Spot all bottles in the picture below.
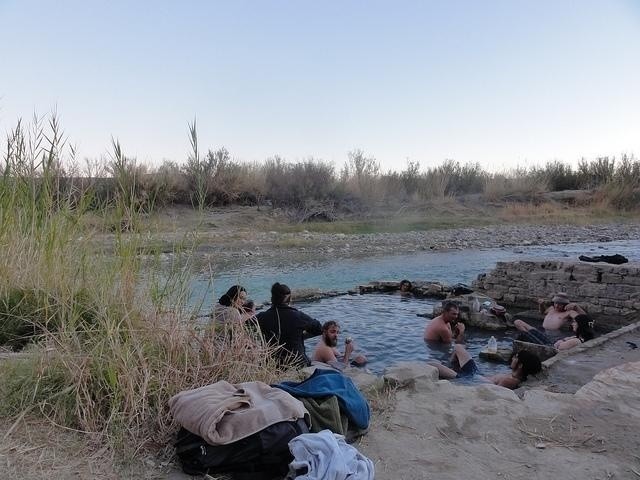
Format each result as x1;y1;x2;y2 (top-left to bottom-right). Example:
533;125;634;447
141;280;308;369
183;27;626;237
487;335;498;355
472;298;480;313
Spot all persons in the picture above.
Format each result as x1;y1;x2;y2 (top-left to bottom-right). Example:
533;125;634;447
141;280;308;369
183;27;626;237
516;314;594;351
513;291;587;334
239;299;257;321
313;320;355;365
422;301;466;344
426;342;542;390
393;280;414;296
199;282;248;354
245;282;325;373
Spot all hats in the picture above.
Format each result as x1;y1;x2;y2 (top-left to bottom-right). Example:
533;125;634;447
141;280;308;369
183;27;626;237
551;291;570;304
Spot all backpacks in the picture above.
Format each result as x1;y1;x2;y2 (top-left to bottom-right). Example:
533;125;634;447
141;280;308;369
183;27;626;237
174;416;314;480
